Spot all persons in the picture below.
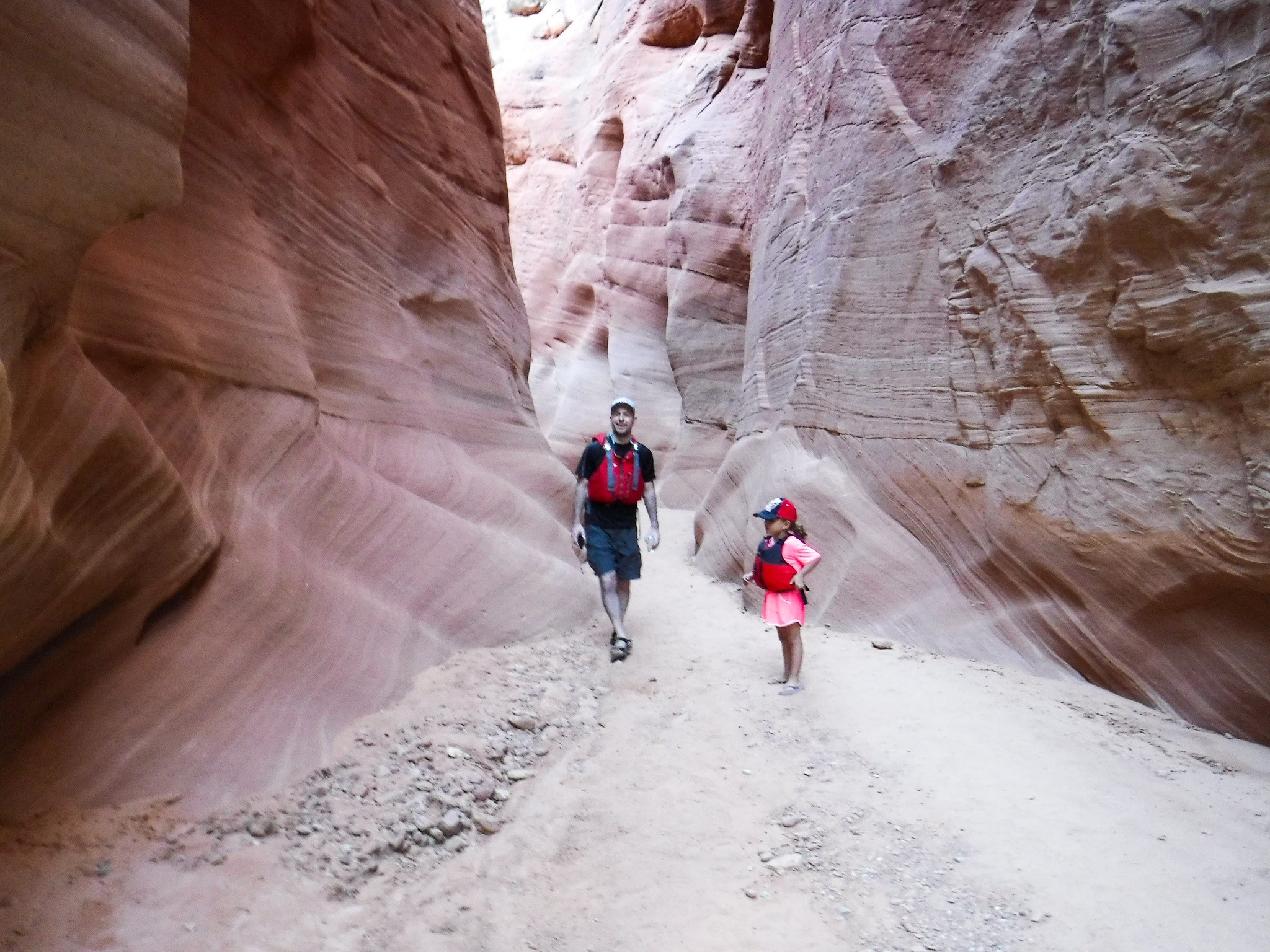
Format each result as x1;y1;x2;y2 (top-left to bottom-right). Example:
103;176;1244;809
743;498;823;697
571;397;660;662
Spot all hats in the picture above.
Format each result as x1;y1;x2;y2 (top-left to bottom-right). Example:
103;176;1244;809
753;497;797;520
610;397;635;414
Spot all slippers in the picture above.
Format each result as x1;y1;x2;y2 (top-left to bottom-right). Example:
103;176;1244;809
778;684;804;696
768;678;787;685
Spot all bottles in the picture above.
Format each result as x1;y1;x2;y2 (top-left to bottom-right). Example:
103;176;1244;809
646;535;655;553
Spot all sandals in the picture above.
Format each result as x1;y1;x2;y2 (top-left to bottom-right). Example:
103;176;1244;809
610;636;632;662
610;631;616;646
